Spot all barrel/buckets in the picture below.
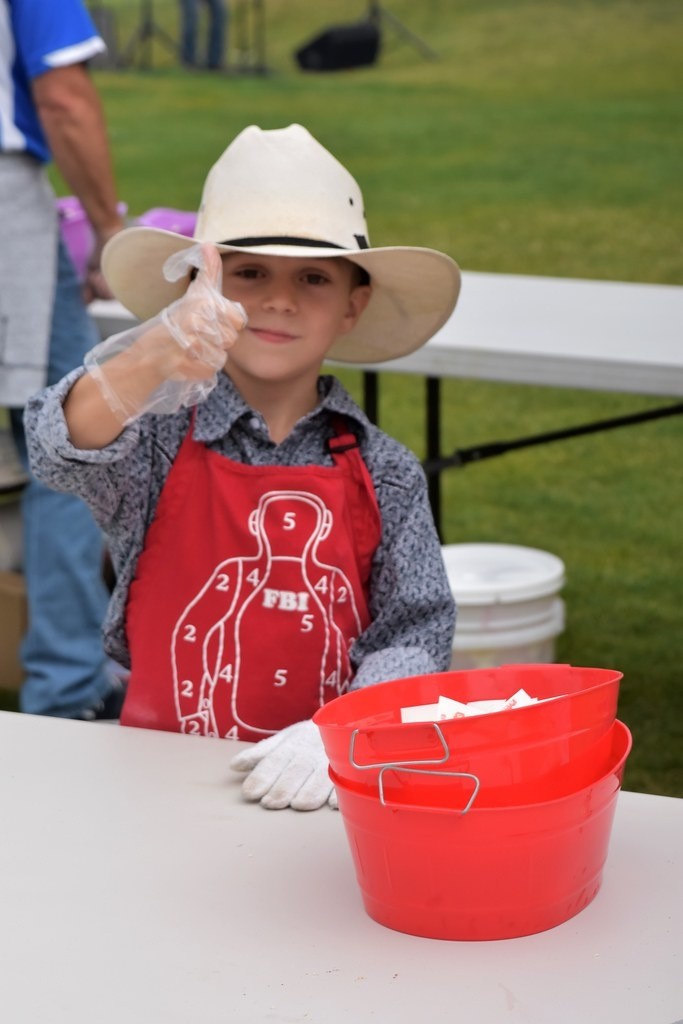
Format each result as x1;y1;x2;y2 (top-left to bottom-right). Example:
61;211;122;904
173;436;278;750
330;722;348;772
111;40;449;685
312;541;637;943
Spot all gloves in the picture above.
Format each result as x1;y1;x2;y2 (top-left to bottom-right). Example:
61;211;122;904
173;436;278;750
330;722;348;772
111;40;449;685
229;718;341;810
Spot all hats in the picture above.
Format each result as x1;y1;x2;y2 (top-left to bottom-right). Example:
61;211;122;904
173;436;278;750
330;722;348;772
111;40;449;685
100;123;463;365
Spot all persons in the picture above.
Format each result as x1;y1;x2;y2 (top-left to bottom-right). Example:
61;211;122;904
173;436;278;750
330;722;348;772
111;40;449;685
20;122;461;813
0;0;129;718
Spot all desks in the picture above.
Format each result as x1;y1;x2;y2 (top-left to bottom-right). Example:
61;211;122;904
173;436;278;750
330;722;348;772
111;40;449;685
82;266;682;553
0;709;682;1020
